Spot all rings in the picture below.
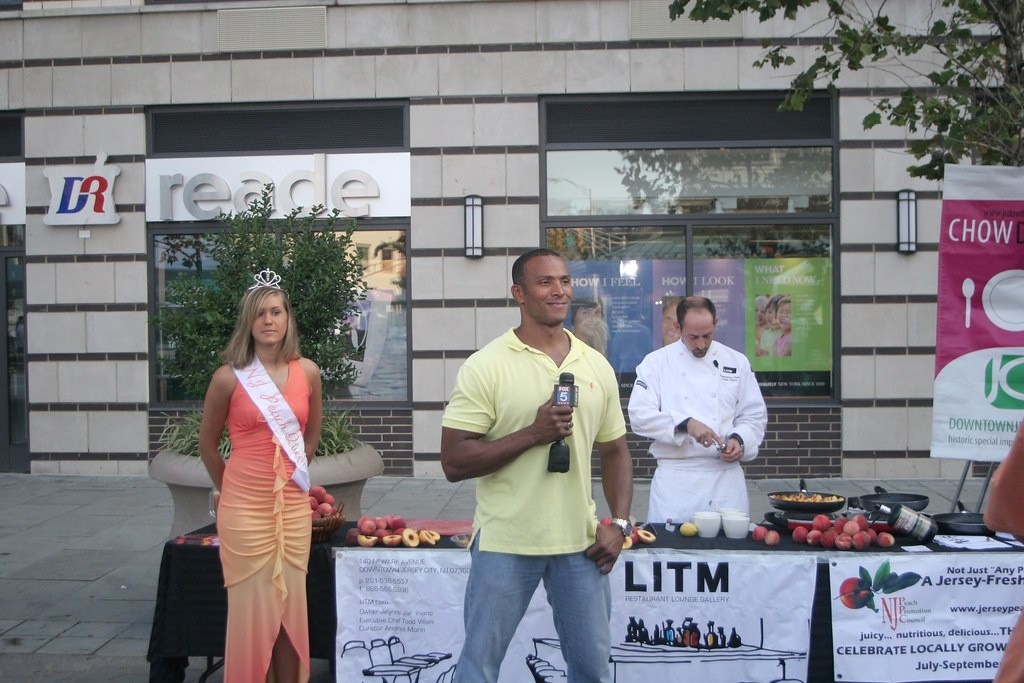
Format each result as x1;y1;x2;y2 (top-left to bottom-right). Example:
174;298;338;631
567;422;573;430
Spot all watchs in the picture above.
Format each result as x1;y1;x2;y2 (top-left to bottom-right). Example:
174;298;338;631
609;518;633;536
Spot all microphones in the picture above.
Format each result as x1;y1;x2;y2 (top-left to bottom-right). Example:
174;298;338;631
547;371;579;472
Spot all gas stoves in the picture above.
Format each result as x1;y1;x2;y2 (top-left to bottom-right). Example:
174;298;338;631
763;511;932;532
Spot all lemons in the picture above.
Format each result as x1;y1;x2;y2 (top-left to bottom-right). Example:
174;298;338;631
680;522;698;537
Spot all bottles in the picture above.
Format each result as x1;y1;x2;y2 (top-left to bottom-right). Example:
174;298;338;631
875;504;938;545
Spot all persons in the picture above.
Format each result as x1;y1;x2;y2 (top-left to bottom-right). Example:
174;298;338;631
981;419;1024;683
628;295;768;524
441;249;634;683
662;295;683;347
755;294;792;357
569;297;606;358
200;267;322;683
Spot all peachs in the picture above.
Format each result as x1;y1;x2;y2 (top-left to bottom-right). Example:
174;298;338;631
309;485;337;520
753;525;780;545
599;517;655;550
792;514;894;551
839;577;869;609
345;515;440;547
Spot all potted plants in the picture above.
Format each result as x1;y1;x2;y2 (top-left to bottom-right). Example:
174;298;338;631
147;181;384;540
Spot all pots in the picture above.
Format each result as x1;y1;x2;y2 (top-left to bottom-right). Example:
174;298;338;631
859;486;930;513
933;501;987;533
767;479;846;512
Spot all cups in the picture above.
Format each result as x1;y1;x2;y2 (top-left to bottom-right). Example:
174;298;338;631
694;512;721;538
209;486;221;522
720;508;750;538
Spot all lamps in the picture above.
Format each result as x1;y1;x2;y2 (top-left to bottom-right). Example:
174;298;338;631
463;194;485;260
896;188;917;256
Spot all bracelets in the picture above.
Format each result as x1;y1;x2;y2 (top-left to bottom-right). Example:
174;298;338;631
729;433;744;450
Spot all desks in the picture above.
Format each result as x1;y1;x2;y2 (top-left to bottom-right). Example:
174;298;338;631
145;521;1024;683
